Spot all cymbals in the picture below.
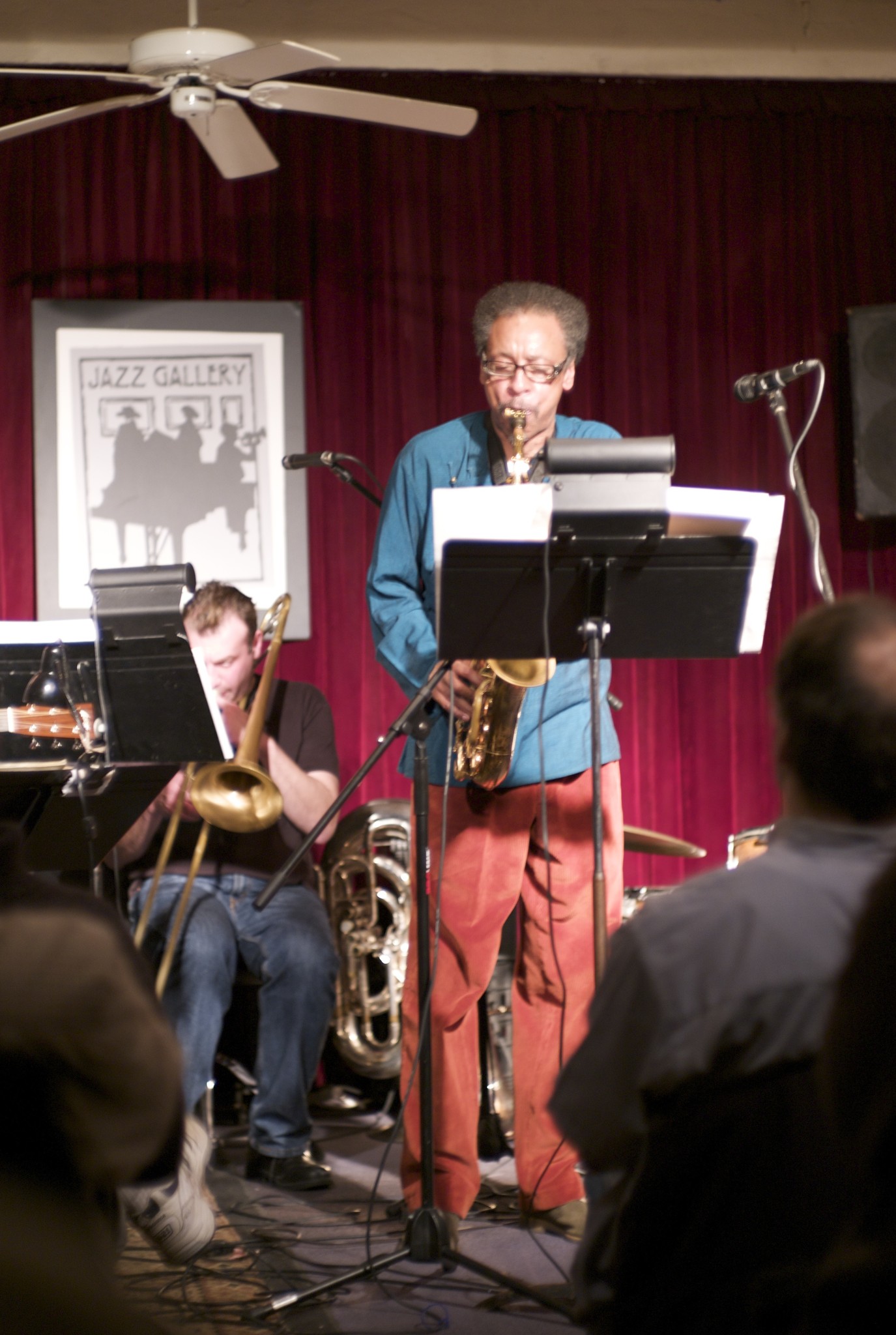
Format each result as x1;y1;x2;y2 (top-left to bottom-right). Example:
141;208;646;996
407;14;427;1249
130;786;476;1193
623;823;707;858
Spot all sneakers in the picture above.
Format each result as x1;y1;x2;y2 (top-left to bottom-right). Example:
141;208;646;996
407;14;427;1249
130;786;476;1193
123;1116;215;1267
246;1140;332;1190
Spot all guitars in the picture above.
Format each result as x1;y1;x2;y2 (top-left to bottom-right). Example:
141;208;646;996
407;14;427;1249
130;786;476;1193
0;698;107;742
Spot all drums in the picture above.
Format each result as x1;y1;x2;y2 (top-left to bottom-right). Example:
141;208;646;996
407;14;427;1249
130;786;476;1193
725;824;775;870
620;885;678;921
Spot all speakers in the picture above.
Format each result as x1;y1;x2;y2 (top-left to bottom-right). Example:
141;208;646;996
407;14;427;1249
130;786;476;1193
844;303;896;519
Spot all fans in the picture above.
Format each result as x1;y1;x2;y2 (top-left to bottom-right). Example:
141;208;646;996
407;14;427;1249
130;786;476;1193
0;0;478;178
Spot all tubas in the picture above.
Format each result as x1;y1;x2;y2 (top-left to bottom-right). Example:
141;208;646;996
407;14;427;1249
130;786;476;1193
312;797;417;1079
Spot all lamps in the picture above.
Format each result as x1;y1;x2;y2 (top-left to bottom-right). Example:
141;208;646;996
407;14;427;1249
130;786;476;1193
172;85;216;137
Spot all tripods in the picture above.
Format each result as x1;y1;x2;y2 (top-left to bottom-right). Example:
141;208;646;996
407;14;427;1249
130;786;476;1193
246;660;582;1323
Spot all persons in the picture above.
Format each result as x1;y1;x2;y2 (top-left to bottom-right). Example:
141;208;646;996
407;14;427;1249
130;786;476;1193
0;823;214;1335
540;590;896;1335
101;582;342;1192
366;281;627;1253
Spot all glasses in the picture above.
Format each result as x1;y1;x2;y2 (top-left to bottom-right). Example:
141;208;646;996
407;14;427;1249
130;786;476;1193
482;344;572;383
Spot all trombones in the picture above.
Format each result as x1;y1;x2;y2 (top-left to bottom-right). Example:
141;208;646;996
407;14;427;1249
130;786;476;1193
128;590;296;1005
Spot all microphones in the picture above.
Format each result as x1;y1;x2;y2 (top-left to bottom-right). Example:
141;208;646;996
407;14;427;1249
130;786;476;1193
281;451;345;471
734;359;819;402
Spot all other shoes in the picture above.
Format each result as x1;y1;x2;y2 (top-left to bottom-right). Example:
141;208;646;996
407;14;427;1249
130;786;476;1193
529;1193;586;1243
402;1198;458;1261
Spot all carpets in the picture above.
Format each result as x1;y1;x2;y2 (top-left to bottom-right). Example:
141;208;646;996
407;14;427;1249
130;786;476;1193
113;1164;337;1334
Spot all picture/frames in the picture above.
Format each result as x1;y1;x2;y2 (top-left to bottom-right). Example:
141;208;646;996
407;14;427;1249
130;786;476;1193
831;296;896;554
30;299;311;643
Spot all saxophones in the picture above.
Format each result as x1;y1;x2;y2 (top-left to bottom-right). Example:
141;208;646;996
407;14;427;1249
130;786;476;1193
455;407;557;791
